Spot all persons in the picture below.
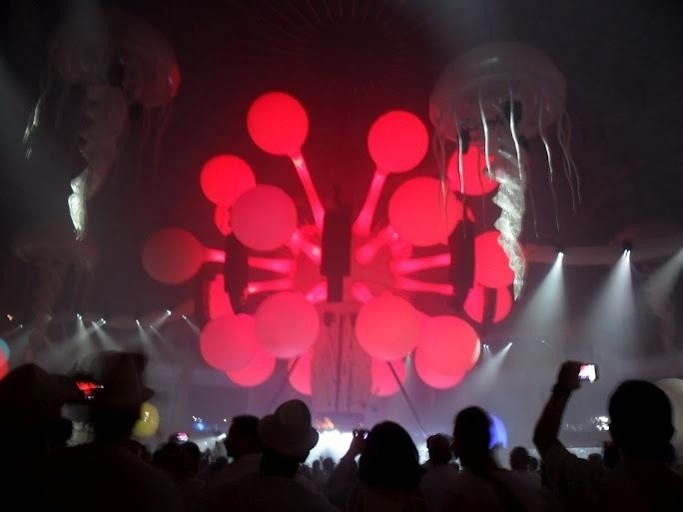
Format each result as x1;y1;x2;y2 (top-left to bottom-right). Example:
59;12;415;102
0;350;683;512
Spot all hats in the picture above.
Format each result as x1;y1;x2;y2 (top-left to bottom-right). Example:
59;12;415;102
88;352;154;404
257;400;318;454
0;363;75;412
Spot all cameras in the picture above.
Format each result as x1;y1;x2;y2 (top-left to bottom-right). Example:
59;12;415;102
578;363;599;383
